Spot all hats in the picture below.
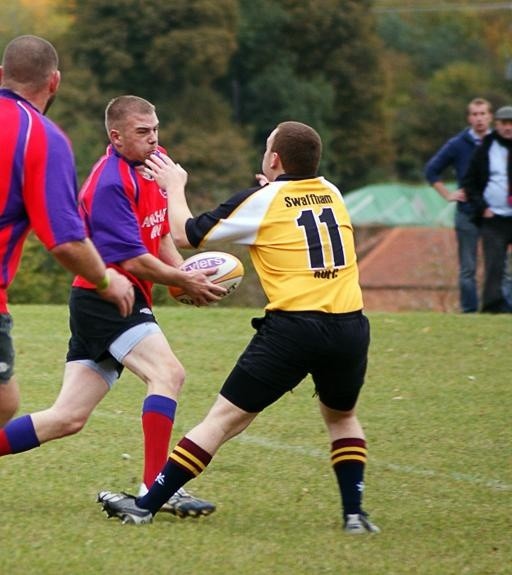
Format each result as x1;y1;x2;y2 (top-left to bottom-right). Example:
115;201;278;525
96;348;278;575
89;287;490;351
494;105;512;120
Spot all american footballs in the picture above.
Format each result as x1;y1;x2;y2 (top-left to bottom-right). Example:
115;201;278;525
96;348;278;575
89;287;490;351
167;251;246;307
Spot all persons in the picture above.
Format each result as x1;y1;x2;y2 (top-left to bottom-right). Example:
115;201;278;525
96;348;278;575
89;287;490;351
425;97;494;312
2;96;227;518
97;121;381;535
1;36;135;432
463;106;512;313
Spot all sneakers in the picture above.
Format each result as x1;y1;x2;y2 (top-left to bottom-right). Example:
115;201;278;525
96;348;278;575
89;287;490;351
140;483;216;517
97;489;155;526
344;511;382;535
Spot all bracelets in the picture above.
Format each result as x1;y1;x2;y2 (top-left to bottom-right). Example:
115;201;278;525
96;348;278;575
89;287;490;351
97;270;109;291
